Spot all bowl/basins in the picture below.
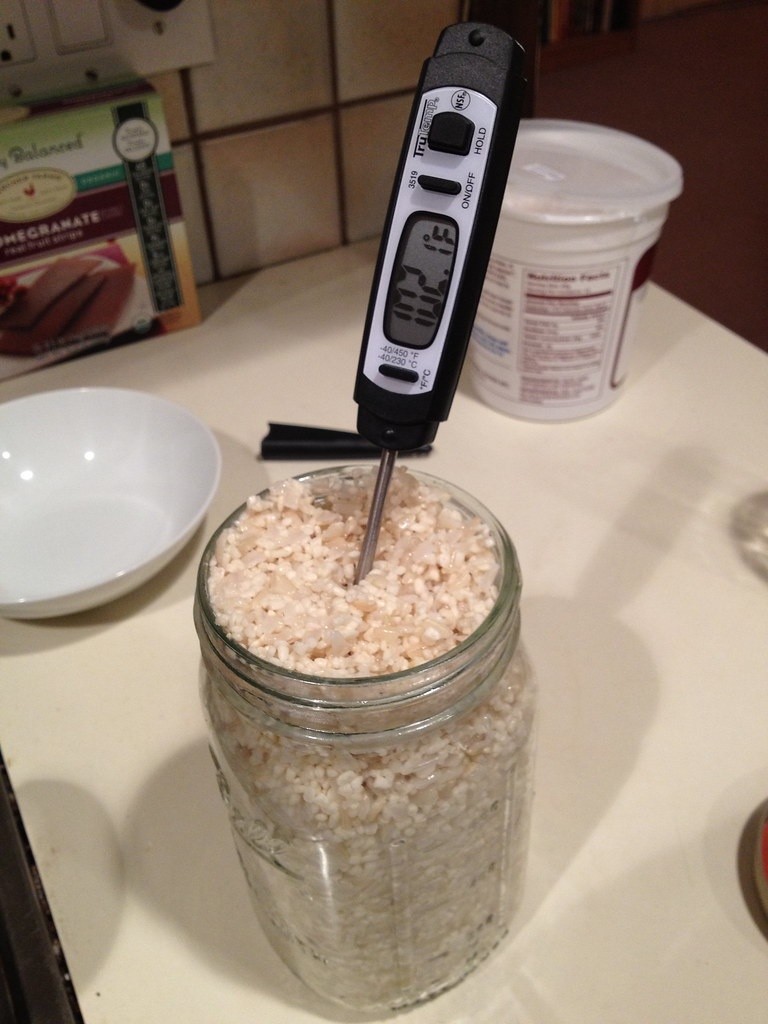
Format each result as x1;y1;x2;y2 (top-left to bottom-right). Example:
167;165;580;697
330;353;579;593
0;386;223;621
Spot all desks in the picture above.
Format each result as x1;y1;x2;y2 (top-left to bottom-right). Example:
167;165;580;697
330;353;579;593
0;231;768;1024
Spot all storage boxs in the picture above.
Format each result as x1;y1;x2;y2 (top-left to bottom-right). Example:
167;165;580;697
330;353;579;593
0;81;203;387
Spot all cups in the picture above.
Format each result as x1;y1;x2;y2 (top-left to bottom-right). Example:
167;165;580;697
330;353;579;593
462;113;684;423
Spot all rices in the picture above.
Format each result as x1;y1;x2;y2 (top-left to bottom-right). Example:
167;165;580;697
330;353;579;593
200;464;536;1011
